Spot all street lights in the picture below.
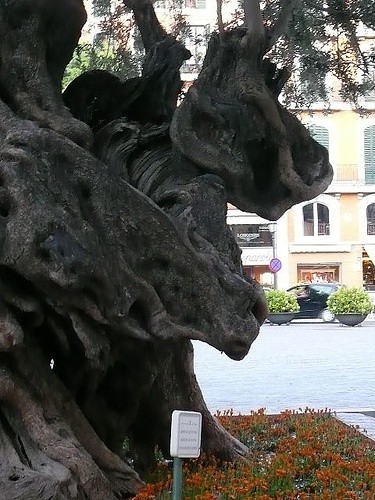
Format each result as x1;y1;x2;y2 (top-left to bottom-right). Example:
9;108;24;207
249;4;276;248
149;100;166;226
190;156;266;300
267;219;279;289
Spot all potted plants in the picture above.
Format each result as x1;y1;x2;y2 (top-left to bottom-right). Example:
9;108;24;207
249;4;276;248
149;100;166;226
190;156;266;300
266;290;301;326
326;286;372;326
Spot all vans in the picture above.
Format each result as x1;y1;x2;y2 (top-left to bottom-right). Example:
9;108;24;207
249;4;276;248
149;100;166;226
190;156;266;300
283;284;347;324
